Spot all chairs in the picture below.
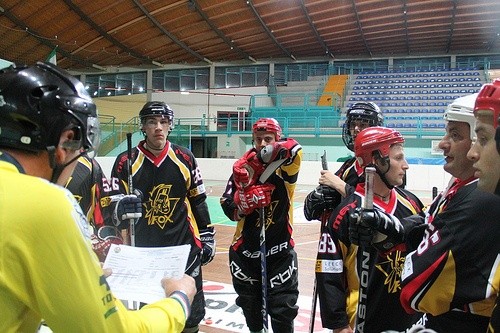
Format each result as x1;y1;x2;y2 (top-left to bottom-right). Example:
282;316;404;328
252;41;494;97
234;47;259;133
345;67;482;129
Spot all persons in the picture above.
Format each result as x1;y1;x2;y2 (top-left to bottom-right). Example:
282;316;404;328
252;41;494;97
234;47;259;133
349;94;500;333
221;117;303;333
61;154;115;238
315;127;423;333
0;61;197;333
466;76;500;200
305;102;384;222
110;100;217;333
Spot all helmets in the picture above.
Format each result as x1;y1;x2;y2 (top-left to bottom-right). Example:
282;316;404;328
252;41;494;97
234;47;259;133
138;101;175;122
474;80;500;126
444;91;480;143
342;102;384;152
354;125;405;165
0;62;96;153
252;117;281;141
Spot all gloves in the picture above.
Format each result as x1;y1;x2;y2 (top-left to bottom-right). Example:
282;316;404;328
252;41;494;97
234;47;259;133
233;184;274;214
349;207;407;254
110;190;144;228
198;229;215;266
232;151;266;189
305;186;337;220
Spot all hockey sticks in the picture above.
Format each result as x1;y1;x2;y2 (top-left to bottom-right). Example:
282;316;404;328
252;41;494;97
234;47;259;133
308;209;327;333
126;132;137;311
260;205;269;333
354;166;376;333
